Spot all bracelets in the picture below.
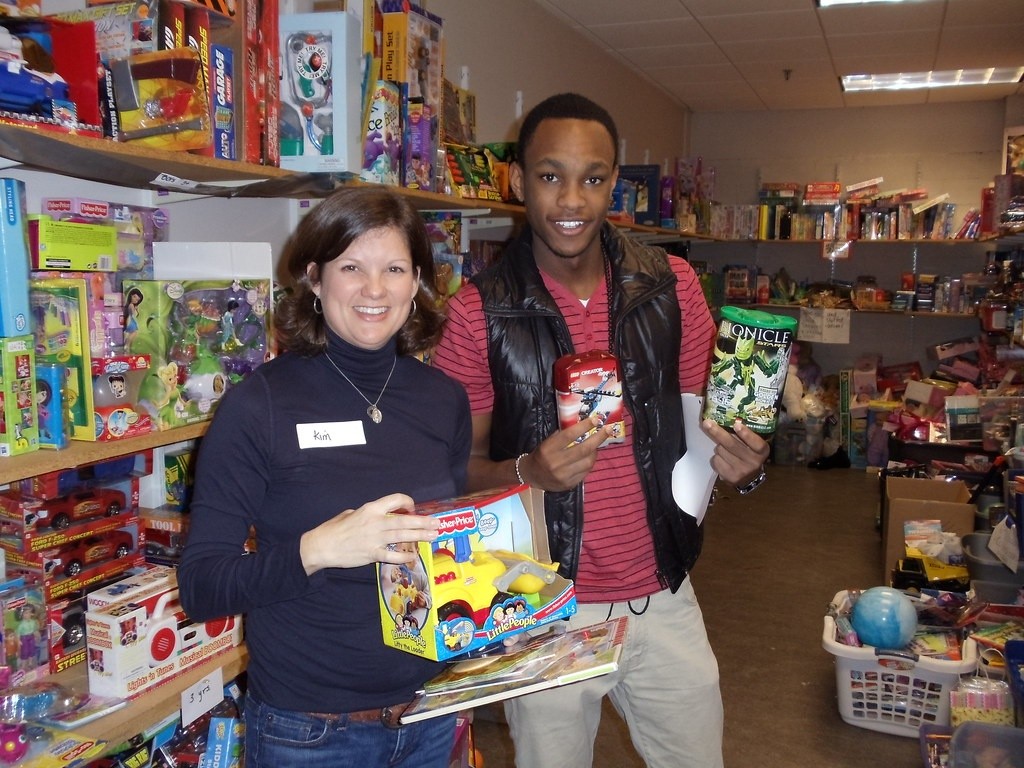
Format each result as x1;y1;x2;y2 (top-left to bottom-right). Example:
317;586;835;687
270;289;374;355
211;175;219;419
516;452;529;485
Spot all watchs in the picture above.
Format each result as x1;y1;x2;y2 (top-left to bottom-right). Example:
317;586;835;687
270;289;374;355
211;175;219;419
737;465;766;497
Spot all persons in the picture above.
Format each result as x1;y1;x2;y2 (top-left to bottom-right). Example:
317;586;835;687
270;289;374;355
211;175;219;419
430;89;770;768
383;542;432;610
178;190;472;768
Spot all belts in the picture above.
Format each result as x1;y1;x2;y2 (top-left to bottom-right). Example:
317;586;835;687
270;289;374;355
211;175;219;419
293;700;412;729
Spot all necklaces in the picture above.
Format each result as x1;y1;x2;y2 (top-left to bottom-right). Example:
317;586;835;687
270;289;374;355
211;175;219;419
600;244;615;353
324;350;397;425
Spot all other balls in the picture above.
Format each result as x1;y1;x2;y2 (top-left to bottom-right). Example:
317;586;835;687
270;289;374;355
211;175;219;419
850;585;918;650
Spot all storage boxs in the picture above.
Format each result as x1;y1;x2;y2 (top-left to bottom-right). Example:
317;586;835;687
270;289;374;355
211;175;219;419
0;0;1024;768
608;176;1024;768
0;0;576;768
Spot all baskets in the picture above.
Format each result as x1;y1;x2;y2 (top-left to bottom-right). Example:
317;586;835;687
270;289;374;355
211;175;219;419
821;589;977;738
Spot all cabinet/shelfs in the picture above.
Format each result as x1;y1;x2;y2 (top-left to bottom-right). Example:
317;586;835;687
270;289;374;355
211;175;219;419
978;226;1024;348
722;239;978;318
0;116;304;768
